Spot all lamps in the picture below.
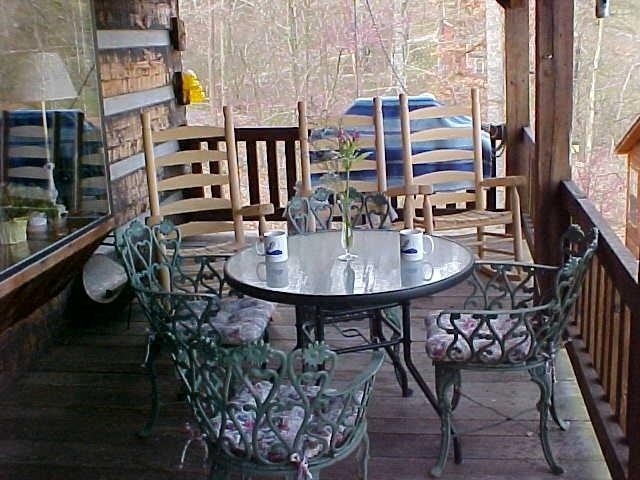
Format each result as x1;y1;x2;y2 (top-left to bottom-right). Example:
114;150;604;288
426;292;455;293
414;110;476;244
9;50;80;224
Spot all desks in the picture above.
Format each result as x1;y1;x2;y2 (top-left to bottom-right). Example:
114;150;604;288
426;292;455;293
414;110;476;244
224;225;477;465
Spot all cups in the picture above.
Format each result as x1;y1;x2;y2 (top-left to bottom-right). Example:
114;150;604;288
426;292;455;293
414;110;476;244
255;262;289;288
400;261;434;287
255;231;288;262
396;229;434;263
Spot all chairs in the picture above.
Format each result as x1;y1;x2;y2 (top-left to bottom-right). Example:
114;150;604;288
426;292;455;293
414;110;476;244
398;88;528;280
141;106;275;329
168;319;384;480
113;216;277;437
294;96;419;233
428;224;600;477
